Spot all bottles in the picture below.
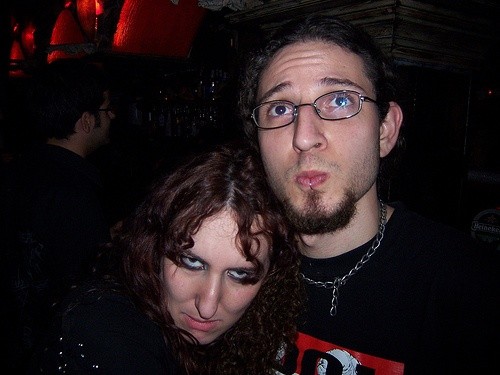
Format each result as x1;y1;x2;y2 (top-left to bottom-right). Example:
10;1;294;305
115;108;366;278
170;68;221;130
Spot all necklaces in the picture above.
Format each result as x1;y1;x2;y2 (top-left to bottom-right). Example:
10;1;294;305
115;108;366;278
298;199;386;318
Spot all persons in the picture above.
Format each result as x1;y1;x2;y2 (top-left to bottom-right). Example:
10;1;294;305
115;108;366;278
0;15;500;375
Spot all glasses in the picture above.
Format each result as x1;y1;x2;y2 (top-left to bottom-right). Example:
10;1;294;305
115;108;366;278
248;90;379;129
94;104;114;115
